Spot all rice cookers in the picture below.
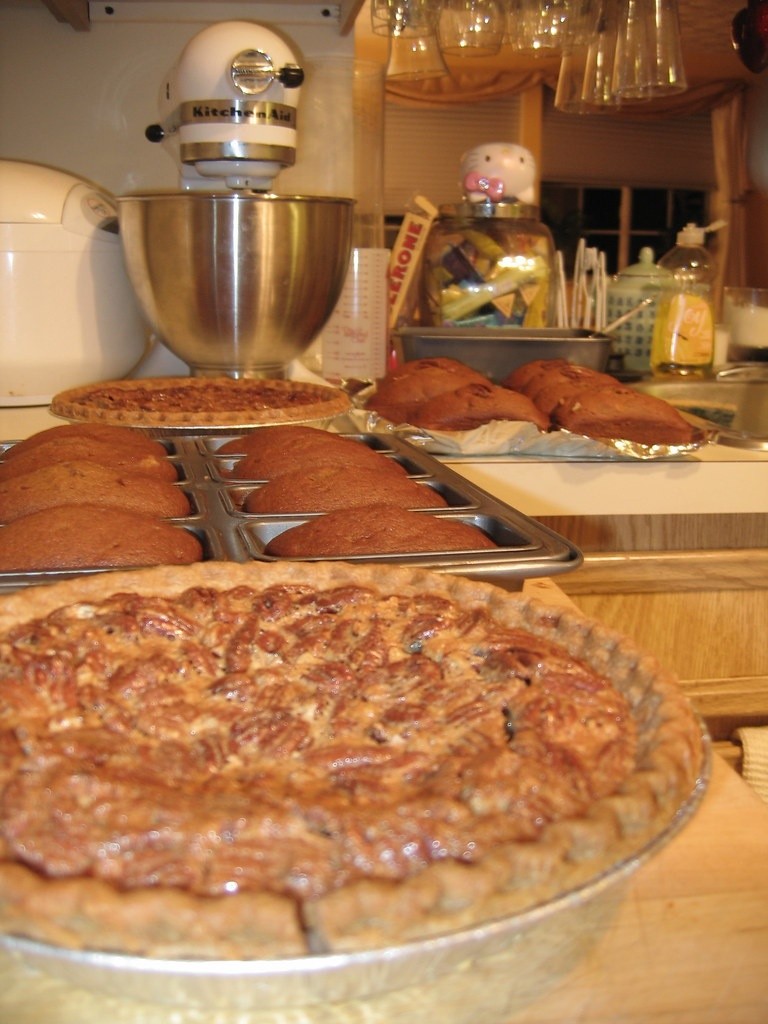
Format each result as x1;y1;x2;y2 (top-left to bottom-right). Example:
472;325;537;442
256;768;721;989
1;160;152;408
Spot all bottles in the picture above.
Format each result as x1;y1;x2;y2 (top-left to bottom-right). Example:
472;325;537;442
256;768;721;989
648;217;735;380
424;202;559;327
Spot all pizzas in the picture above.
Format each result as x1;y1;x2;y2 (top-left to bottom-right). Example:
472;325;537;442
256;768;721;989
0;559;708;956
49;377;354;427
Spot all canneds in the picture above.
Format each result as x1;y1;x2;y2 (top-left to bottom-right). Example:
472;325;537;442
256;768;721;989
421;202;560;331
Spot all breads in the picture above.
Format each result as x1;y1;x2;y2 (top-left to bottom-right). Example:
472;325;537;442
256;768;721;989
363;356;694;445
213;422;499;557
0;419;202;574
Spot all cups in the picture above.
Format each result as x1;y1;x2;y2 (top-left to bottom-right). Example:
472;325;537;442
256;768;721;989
723;285;767;362
369;0;689;115
607;245;675;371
321;248;386;378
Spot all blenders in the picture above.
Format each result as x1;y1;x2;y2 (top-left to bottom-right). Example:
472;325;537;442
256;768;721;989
129;19;337;391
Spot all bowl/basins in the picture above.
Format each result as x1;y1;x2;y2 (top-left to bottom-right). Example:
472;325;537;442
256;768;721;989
388;327;612;387
111;191;357;383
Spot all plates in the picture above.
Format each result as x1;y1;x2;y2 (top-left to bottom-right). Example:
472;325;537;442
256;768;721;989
50;403;358;441
1;704;708;1011
